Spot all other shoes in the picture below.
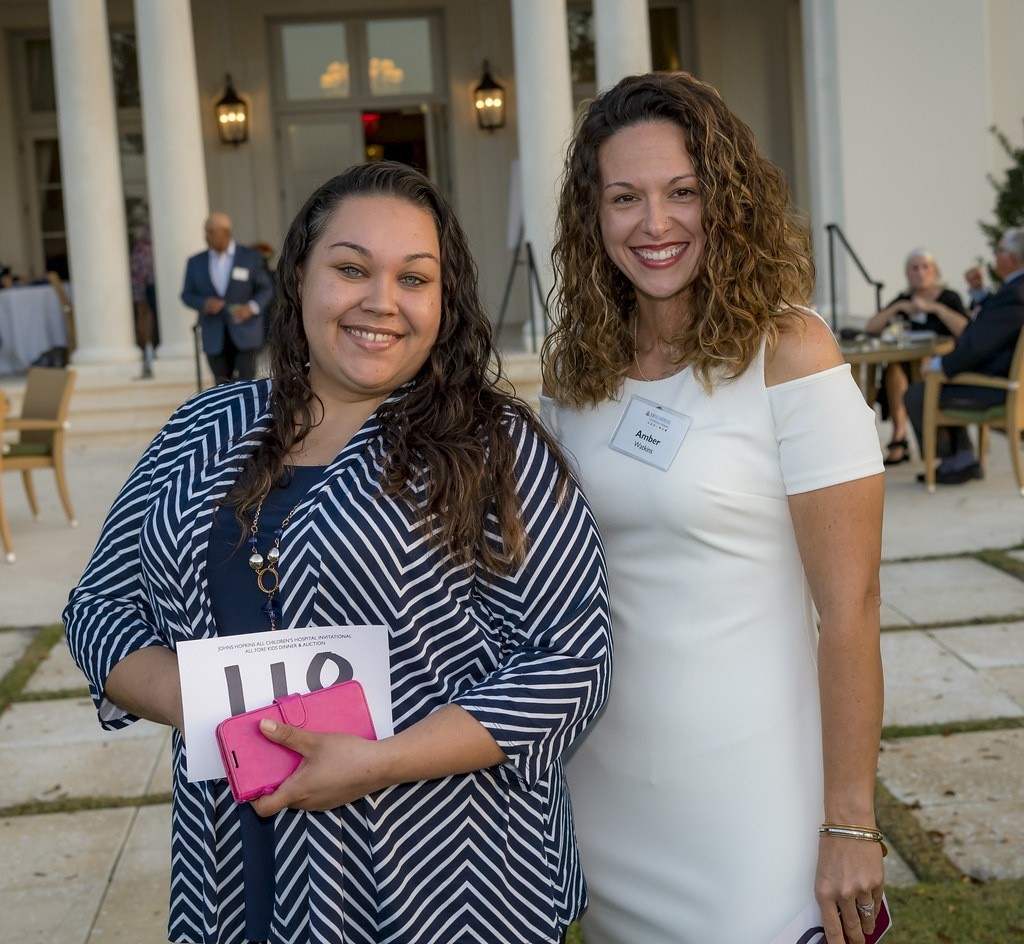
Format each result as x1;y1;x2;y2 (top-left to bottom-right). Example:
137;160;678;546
883;439;910;465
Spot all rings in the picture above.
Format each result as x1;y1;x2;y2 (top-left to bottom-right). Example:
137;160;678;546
856;898;875;916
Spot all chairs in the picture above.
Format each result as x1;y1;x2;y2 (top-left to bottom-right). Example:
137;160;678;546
920;325;1024;497
0;368;78;528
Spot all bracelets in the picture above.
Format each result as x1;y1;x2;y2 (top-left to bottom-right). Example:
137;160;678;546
819;823;888;856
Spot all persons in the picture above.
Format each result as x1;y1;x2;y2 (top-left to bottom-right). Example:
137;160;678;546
180;211;273;385
865;248;971;465
903;227;1024;486
59;164;613;944
537;68;891;944
130;230;159;349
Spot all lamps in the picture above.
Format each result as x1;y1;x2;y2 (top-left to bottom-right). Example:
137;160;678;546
215;73;250;150
474;58;507;134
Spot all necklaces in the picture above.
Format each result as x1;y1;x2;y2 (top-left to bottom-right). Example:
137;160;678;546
634;304;680;381
248;471;305;596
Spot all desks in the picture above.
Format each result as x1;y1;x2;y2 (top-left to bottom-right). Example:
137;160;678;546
836;333;955;406
0;280;73;377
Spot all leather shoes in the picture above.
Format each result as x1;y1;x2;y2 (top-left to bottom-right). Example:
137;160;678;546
917;460;983;485
